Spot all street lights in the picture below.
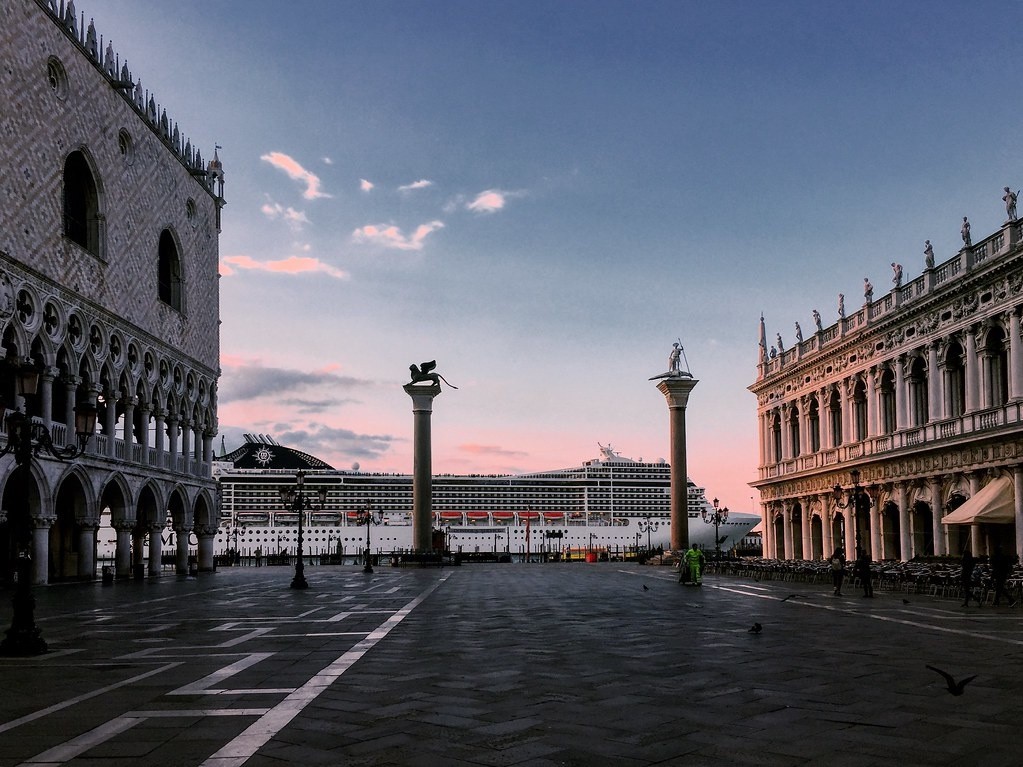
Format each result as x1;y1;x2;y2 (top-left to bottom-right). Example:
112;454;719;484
701;497;729;570
356;497;384;573
280;468;327;590
637;515;659;550
225;515;245;557
0;355;97;658
832;466;879;584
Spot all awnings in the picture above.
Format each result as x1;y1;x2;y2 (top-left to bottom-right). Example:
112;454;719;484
941;476;1015;523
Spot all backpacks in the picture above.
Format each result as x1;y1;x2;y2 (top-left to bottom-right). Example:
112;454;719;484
831;555;843;571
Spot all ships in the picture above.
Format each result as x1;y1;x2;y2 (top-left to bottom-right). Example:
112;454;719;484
94;434;761;556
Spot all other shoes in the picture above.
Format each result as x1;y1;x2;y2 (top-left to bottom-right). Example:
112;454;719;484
979;600;982;608
834;590;840;595
960;604;969;607
989;601;1000;607
1008;600;1018;608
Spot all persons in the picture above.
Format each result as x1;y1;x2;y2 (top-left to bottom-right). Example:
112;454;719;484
989;545;1018;607
854;549;872;598
226;547;234;557
827;547;846;595
684;543;705;585
956;549;978;607
253;547;262;567
280;548;289;562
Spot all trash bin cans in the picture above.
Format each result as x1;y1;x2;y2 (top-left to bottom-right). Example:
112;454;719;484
132;564;145;581
102;566;114;584
372;554;378;566
189;561;199;576
320;554;329;565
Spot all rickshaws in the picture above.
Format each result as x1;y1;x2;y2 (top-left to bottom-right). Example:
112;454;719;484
677;558;706;585
